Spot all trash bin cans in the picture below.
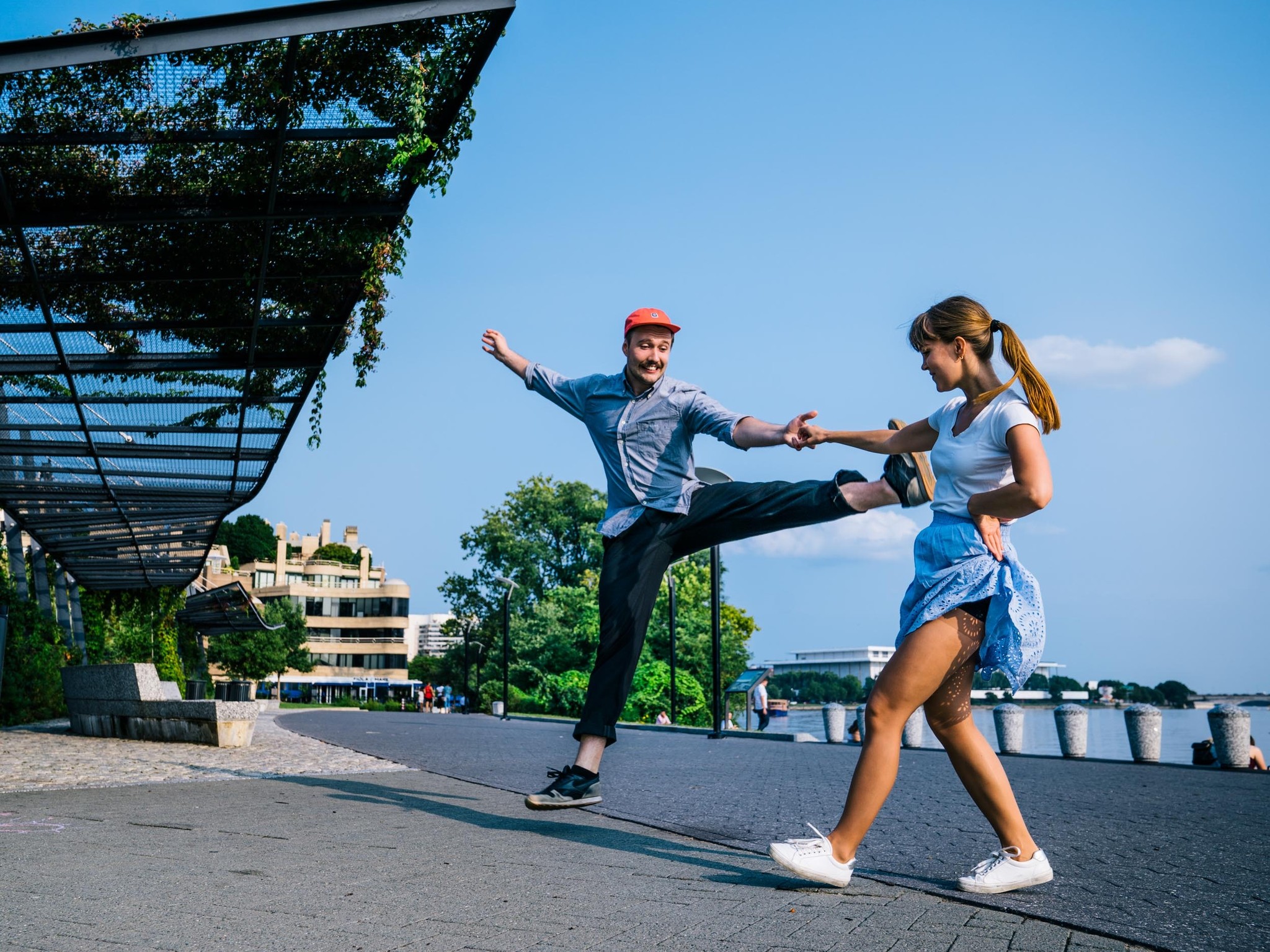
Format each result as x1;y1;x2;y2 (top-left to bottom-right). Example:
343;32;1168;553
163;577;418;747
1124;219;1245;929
215;681;231;701
492;701;504;714
186;680;208;700
232;681;251;701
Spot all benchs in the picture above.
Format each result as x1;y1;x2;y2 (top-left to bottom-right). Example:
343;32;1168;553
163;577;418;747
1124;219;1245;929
59;662;260;747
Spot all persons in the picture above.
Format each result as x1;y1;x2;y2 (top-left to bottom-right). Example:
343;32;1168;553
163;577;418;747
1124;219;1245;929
656;711;671;725
436;684;444;714
480;308;936;809
753;678;770;731
1250;735;1267;770
442;684;452;714
1191;738;1220;766
767;294;1061;894
418;688;424;712
424;682;435;713
721;713;739;730
848;720;861;742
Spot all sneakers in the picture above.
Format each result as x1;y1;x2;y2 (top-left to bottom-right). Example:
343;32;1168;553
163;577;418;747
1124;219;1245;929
880;419;936;508
770;822;859;890
527;765;603;809
958;846;1054;894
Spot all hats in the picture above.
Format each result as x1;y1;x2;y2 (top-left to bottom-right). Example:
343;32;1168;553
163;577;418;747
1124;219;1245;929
625;308;681;335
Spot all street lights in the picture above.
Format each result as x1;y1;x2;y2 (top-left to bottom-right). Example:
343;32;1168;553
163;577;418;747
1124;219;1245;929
473;639;485;710
663;555;690;726
493;576;522;721
458;615;478;713
